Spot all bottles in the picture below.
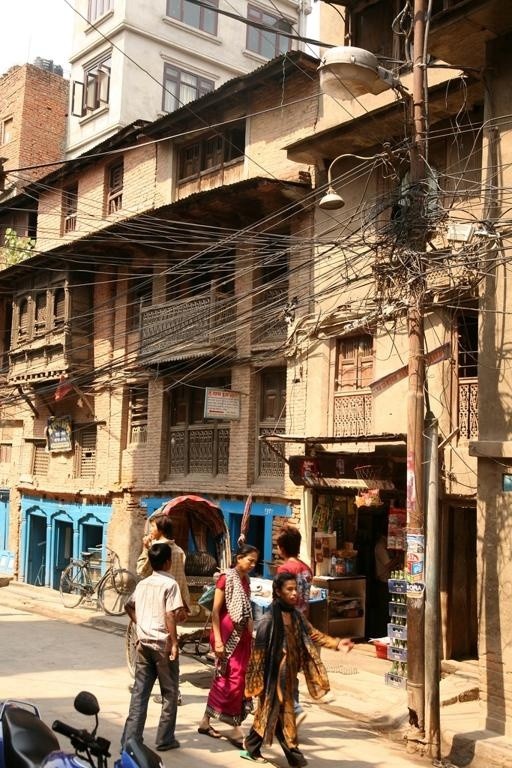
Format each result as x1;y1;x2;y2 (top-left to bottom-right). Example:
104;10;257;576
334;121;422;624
388;570;407;679
335;556;353;577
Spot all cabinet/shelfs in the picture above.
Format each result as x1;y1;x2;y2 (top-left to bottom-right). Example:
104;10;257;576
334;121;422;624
313;575;367;639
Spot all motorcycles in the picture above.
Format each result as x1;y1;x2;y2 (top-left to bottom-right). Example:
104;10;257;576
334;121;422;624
0;691;169;767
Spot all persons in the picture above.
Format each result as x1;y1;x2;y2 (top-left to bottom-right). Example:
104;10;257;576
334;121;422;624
120;514;190;754
198;525;355;767
372;534;402;636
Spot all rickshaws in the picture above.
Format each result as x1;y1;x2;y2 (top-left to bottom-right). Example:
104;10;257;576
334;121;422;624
124;491;279;687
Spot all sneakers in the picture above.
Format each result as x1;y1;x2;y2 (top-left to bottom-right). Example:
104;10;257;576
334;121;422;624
295;712;307;727
129;684;134;693
154;696;181;705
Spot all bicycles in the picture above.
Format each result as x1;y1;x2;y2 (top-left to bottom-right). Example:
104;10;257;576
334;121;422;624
58;541;139;617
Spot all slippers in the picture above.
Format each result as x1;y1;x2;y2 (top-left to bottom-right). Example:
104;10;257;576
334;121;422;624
198;726;222;738
228;736;267;763
157;740;180;750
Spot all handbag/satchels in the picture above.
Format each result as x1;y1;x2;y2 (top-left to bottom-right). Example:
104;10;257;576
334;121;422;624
197;586;215;611
